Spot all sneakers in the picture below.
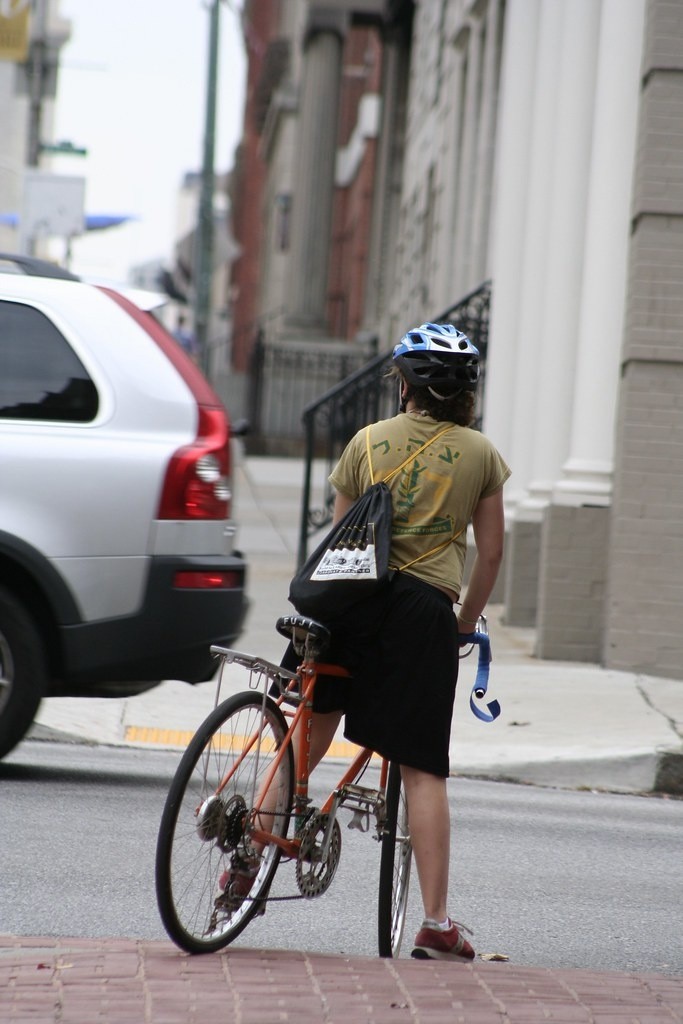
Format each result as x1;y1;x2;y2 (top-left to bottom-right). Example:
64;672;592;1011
220;864;267;915
411;917;475;963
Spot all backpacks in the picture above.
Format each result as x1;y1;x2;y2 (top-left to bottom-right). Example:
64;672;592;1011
288;423;467;626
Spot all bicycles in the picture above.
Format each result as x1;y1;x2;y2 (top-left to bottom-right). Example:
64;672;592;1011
152;611;494;961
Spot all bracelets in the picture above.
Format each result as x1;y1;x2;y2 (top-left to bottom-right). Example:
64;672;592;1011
459;612;479;625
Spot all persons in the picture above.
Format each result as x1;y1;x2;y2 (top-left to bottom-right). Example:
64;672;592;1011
218;321;512;963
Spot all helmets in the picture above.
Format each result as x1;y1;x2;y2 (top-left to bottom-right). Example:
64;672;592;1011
391;322;479;395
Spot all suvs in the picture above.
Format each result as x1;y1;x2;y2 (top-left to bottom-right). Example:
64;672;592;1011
0;269;251;777
